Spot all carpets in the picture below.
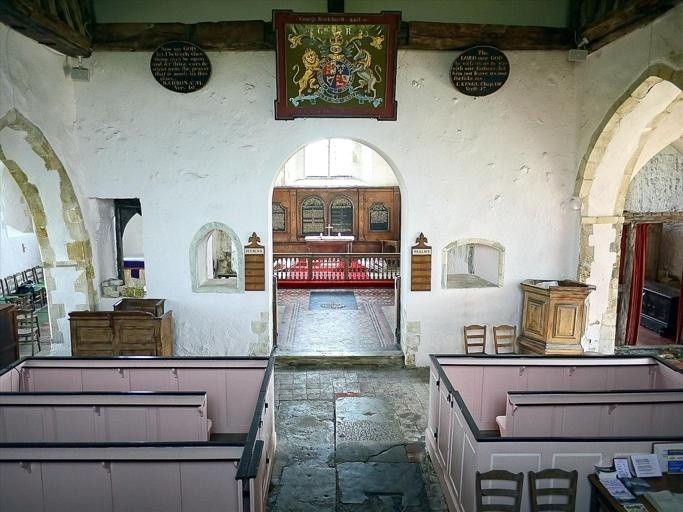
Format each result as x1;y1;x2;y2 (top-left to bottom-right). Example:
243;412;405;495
277;286;395;350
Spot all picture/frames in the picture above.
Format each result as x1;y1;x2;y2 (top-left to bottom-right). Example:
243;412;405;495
274;9;402;122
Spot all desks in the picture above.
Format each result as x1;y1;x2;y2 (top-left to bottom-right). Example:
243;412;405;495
588;472;683;512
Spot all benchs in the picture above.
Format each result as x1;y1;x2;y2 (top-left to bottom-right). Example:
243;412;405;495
496;388;683;437
0;390;212;443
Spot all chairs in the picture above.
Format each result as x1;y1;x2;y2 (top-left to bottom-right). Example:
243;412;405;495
493;325;517;355
1;264;46;367
475;468;525;511
464;325;488;355
528;469;578;509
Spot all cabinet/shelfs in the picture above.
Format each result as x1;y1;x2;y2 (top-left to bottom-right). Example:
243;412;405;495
640;280;679;337
272;186;400;257
68;299;173;360
515;278;596;356
